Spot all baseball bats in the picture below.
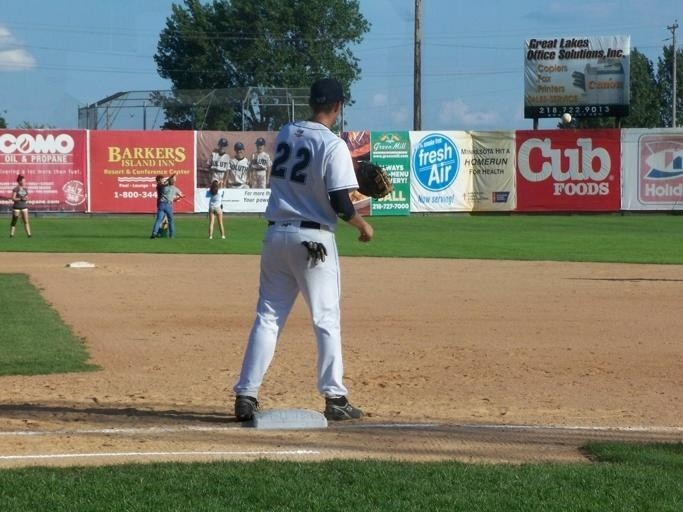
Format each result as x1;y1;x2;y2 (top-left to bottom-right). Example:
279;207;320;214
164;172;178;183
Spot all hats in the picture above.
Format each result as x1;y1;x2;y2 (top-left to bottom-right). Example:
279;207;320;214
218;139;228;146
310;79;342;103
234;143;245;149
257;139;267;146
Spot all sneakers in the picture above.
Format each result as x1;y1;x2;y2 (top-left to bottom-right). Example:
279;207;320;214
208;236;225;239
325;395;361;421
235;395;257;421
150;234;155;238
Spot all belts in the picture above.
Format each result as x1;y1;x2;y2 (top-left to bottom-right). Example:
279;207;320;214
300;220;320;229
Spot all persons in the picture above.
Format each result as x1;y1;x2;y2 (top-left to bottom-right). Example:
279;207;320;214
204;137;230;186
206;179;226;240
156;174;181;238
251;137;273;188
9;173;32;237
151;176;186;239
230;141;251;187
229;78;374;423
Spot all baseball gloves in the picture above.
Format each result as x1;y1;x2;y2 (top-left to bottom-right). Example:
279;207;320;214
354;162;393;199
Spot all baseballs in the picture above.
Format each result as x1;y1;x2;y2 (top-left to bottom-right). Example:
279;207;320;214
562;113;571;123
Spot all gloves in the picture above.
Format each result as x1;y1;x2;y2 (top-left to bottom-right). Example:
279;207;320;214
302;240;327;266
